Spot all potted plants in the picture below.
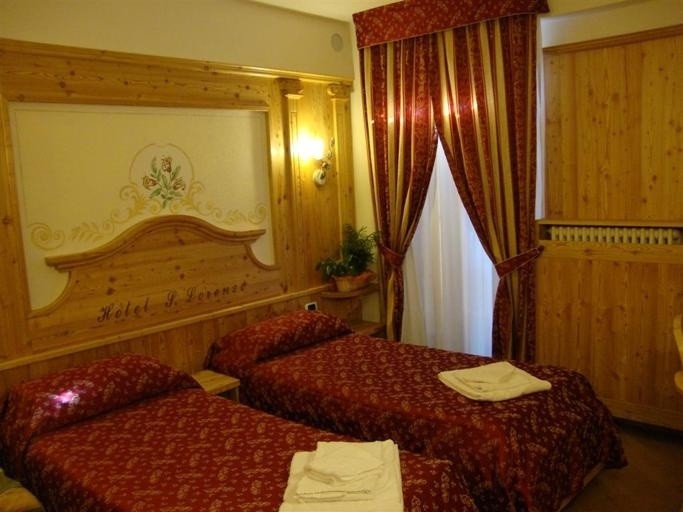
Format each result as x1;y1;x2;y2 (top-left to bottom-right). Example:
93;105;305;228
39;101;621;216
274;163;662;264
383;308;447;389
314;221;388;293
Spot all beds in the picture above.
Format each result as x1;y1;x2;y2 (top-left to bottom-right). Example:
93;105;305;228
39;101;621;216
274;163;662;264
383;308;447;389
214;336;626;510
24;356;483;512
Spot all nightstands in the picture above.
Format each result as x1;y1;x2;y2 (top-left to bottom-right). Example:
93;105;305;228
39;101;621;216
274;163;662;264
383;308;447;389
194;367;243;400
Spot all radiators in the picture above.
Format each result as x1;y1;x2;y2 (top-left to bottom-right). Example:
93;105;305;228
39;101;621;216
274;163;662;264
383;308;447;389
545;226;681;247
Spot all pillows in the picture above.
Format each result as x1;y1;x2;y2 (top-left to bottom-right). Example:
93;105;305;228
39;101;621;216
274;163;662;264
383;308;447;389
1;350;203;479
208;307;351;374
